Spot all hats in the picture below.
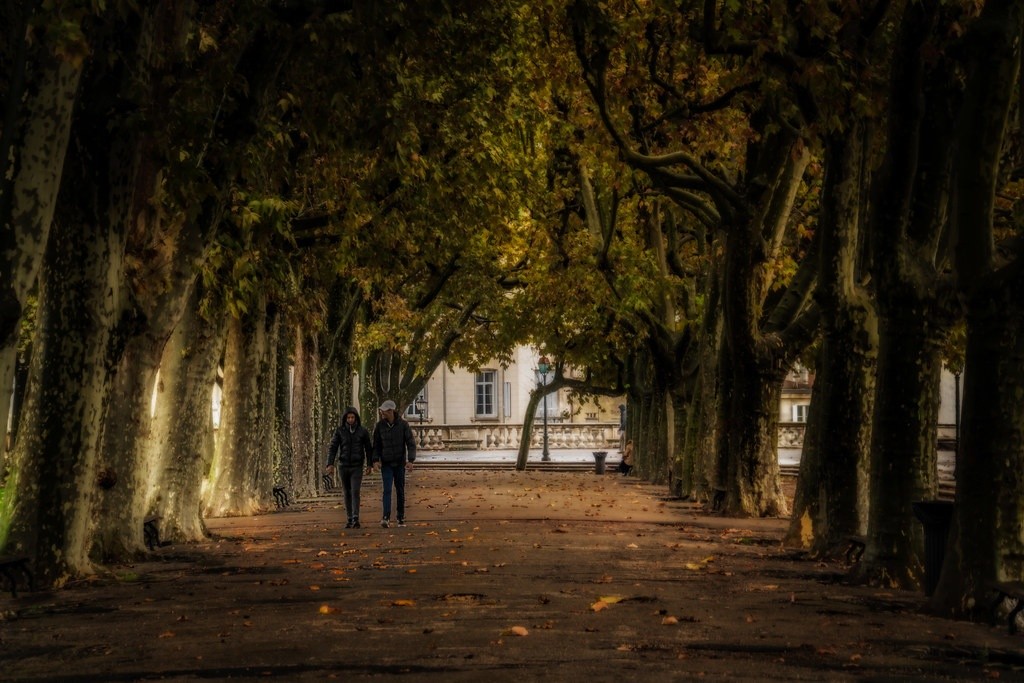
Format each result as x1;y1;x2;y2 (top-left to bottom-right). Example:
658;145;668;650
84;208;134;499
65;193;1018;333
378;400;396;411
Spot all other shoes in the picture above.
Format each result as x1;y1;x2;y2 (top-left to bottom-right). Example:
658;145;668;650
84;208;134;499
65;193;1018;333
381;516;390;527
346;519;352;528
397;518;406;527
353;518;360;528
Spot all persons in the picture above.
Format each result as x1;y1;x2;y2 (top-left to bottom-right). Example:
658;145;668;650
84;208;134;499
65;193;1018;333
326;406;374;528
372;399;417;528
